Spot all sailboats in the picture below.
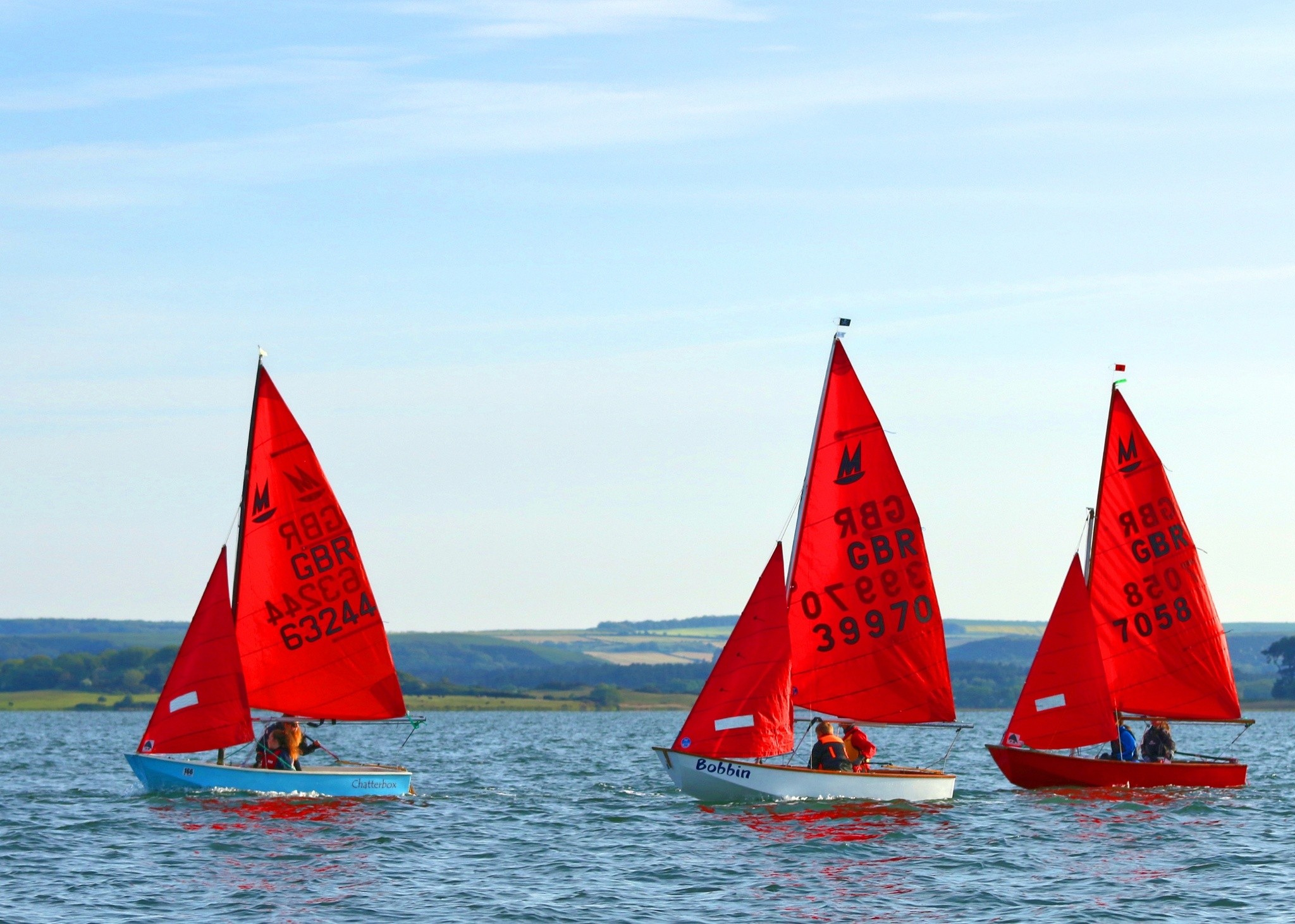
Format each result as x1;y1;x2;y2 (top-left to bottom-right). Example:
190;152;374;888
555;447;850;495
983;361;1257;796
123;340;428;796
647;316;976;805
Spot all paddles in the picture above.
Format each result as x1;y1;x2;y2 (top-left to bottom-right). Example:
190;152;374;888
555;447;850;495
303;734;339;760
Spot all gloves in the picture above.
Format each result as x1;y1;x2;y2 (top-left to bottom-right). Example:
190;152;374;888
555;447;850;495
852;754;866;766
1166;753;1173;761
1143;755;1151;762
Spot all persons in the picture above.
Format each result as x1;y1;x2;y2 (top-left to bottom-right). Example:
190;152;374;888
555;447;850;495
256;711;321;771
807;722;853;773
1101;711;1139;762
1141;719;1176;764
838;717;877;773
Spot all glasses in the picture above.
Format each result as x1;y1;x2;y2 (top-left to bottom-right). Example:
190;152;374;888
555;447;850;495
291;722;299;727
